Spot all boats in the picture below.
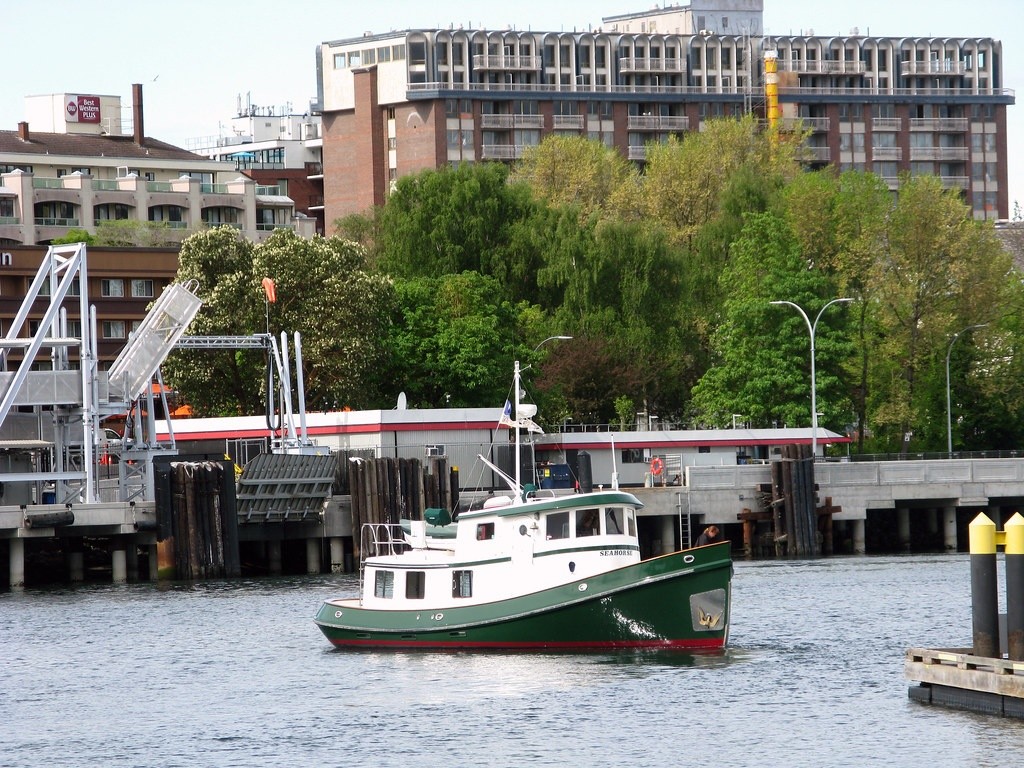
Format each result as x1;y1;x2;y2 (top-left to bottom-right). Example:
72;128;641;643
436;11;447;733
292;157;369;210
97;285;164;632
312;361;735;650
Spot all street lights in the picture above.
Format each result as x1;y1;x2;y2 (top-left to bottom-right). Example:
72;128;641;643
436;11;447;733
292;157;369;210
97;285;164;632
533;336;574;358
771;298;855;461
946;325;987;456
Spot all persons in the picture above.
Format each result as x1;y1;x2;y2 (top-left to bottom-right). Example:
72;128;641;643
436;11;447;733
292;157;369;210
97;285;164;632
694;526;719;548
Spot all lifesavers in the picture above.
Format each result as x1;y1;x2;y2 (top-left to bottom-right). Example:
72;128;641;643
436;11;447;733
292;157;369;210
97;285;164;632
649;457;665;477
